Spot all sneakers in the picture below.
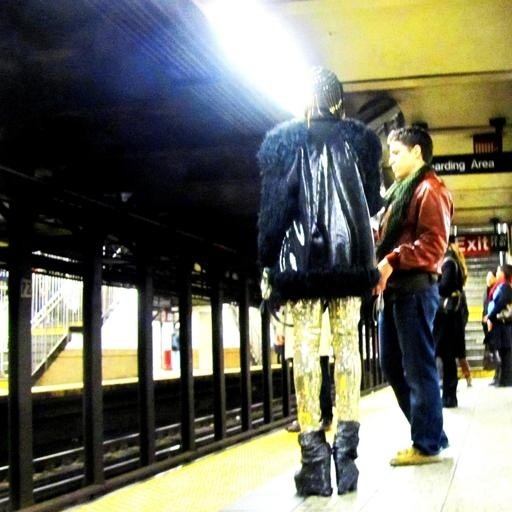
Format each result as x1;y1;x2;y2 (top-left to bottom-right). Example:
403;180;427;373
390;448;441;465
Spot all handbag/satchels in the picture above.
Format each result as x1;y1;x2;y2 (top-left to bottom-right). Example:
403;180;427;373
439;294;463;315
482;350;502;370
496;302;512;323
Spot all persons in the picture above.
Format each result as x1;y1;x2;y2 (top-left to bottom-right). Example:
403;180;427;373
435;234;473;408
480;264;512;387
273;333;285;364
256;66;384;498
369;120;455;468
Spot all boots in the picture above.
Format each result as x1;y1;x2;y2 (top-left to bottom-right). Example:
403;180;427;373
331;420;360;494
294;429;332;497
